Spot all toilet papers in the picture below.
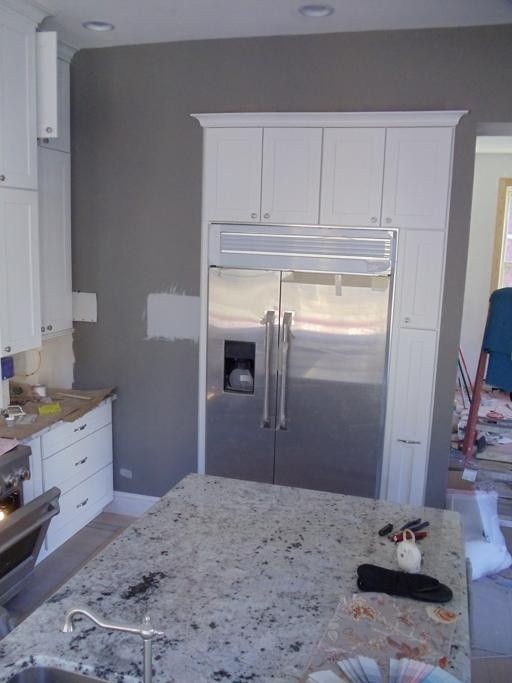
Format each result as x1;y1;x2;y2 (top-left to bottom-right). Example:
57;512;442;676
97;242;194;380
465;490;511;582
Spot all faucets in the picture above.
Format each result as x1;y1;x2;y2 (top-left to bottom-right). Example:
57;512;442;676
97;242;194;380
61;608;164;678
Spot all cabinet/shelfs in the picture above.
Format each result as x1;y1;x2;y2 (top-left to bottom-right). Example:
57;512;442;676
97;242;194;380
379;233;445;506
42;396;115;547
203;124;319;225
34;31;71;150
321;127;454;230
0;27;42;191
2;192;73;354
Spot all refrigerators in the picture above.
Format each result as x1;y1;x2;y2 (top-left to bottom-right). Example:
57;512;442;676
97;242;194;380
205;267;392;500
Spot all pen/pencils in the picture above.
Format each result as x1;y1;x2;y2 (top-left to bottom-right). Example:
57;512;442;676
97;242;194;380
388;518;429;542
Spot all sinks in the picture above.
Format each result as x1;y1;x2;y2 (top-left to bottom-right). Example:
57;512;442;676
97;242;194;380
1;653;142;682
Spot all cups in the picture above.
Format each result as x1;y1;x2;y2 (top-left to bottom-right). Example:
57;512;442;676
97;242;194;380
31;384;46;397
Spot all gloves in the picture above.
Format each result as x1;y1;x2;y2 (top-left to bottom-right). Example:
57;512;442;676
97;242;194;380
357;564;452;603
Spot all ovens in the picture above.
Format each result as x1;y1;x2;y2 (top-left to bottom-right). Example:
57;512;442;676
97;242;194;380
0;446;61;613
